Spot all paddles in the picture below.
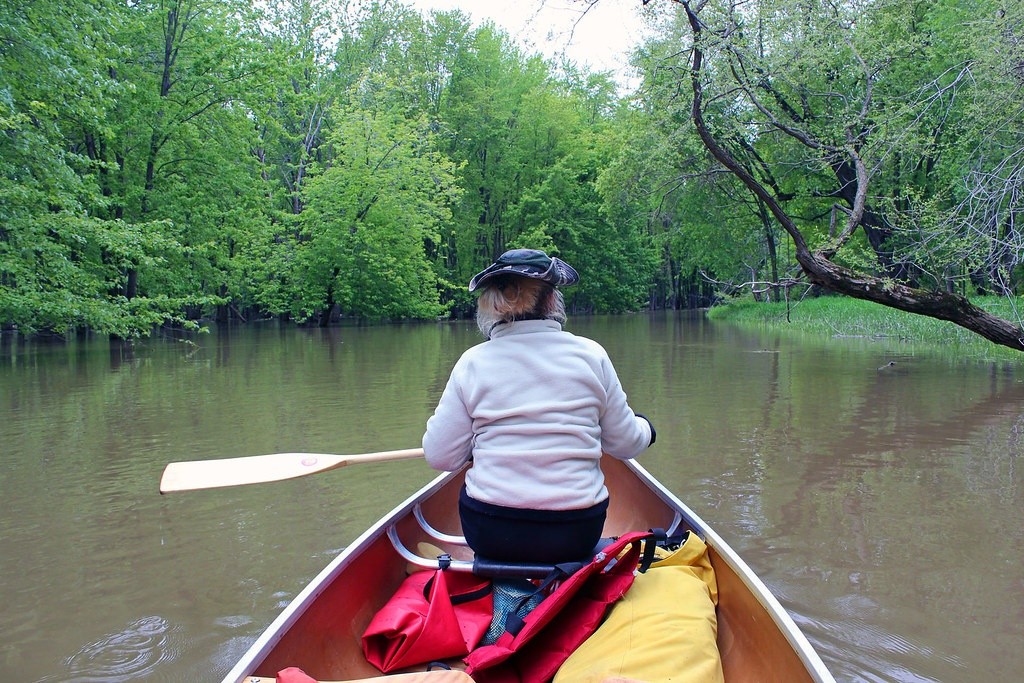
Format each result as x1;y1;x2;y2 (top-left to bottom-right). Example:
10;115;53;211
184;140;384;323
160;445;429;494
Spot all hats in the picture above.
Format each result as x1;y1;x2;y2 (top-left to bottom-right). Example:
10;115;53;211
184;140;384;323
468;249;580;292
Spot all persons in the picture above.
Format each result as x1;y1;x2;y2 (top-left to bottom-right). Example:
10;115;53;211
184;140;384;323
422;250;655;569
